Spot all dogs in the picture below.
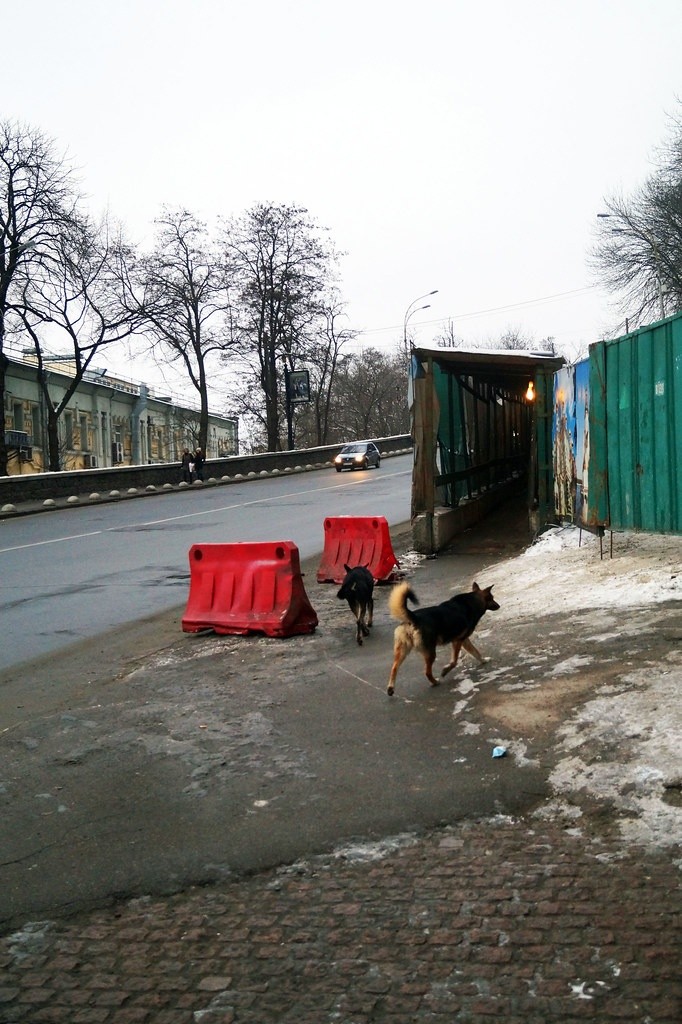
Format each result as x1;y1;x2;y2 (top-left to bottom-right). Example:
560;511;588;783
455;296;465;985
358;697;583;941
336;562;376;646
386;581;500;695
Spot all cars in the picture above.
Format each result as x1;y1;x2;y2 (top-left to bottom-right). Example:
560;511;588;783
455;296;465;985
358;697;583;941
335;442;381;472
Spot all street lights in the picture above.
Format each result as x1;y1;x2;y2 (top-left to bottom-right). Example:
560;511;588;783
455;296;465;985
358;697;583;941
404;290;439;358
610;227;634;231
597;213;665;319
405;305;431;324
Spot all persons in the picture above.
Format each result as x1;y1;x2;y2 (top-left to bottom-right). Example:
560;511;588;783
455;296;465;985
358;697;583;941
194;448;206;482
181;448;194;484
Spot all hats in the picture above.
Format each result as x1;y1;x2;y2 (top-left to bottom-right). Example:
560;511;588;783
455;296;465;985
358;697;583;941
196;447;202;452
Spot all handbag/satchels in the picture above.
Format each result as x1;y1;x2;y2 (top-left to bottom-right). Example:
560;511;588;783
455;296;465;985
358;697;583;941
189;463;194;473
